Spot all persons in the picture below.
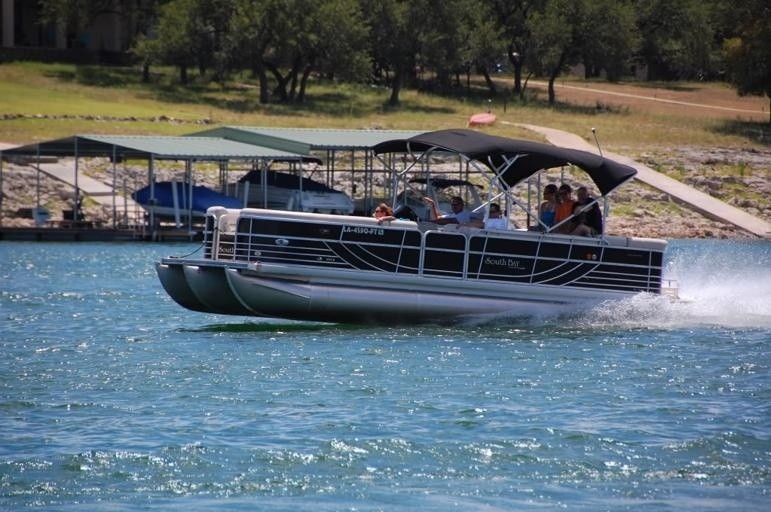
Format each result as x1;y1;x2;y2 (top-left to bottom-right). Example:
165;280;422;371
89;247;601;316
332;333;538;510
525;184;560;233
551;185;575;234
569;187;602;236
372;203;396;225
421;197;484;229
456;203;520;231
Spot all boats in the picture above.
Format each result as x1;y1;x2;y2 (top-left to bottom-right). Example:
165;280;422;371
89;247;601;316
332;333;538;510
129;177;251;228
154;129;697;325
224;169;356;215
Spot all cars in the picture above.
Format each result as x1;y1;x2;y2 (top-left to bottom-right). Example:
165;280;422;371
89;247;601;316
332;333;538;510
391;176;491;227
